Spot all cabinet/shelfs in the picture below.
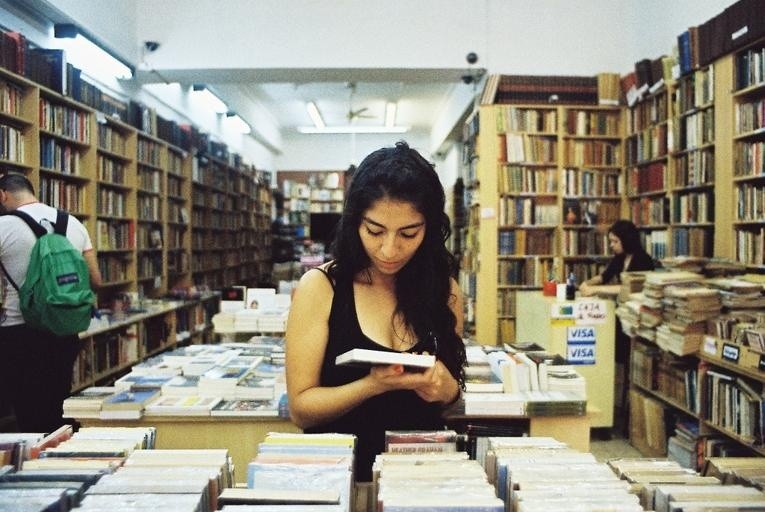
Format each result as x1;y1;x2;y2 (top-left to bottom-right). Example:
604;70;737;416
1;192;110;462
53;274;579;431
38;84;97;261
435;110;489;350
168;143;193;295
175;291;223;348
70;315;141;399
627;59;723;262
139;300;176;363
490;106;627;349
193;150;273;301
723;39;765;271
0;68;38;218
622;324;765;476
94;112;138;311
137;130;168;299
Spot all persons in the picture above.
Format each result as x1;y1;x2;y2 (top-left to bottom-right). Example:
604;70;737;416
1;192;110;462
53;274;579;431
285;141;471;483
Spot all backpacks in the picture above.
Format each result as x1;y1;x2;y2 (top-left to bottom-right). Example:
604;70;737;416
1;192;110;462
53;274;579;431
0;207;95;338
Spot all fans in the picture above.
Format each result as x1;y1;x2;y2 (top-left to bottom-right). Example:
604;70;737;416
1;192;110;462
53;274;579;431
336;82;378;125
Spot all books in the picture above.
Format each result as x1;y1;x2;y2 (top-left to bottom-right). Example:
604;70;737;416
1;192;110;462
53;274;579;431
435;0;765;427
0;24;352;427
3;430;764;511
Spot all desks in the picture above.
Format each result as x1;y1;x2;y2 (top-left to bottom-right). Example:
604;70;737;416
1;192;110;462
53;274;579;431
515;289;618;441
74;348;603;485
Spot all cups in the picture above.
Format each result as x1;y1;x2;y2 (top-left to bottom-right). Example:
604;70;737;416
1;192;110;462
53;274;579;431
543;278;576;301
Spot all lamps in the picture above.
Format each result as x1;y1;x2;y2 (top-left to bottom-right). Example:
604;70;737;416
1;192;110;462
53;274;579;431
226;114;251;135
52;22;132;81
190;83;227;116
